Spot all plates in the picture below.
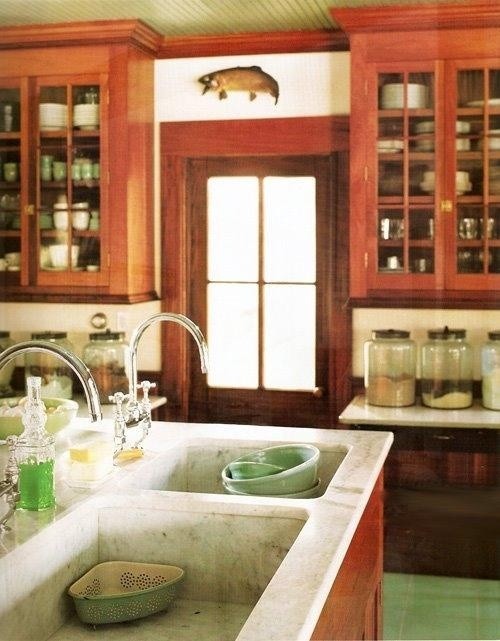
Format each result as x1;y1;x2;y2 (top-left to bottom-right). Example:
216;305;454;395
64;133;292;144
419;171;473;198
466;97;499;104
411;120;473;151
476;128;500;150
379;81;431;109
38;103;100;132
377;138;404;154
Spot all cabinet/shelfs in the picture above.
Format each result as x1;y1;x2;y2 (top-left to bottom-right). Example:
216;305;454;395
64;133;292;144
328;5;500;299
0;18;164;304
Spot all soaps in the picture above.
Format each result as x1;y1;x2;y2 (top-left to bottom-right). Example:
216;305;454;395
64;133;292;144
70;440;112;462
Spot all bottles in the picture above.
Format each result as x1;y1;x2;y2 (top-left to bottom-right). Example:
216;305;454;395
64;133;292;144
0;328;137;405
364;326;499;409
1;193;100;229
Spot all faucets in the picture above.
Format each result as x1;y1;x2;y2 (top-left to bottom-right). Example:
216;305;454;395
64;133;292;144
128;313;210;426
0;341;103;423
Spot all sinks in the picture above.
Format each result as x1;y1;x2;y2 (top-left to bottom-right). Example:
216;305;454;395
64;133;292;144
115;438;353;499
0;494;310;641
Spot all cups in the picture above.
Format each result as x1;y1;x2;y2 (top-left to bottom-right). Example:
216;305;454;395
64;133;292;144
384;250;493;269
379;218;499;238
67;422;111;493
0;242;99;272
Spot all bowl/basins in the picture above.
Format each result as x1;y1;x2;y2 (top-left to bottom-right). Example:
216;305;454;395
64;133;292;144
0;102;19;132
1;154;99;181
0;397;81;440
219;444;322;497
